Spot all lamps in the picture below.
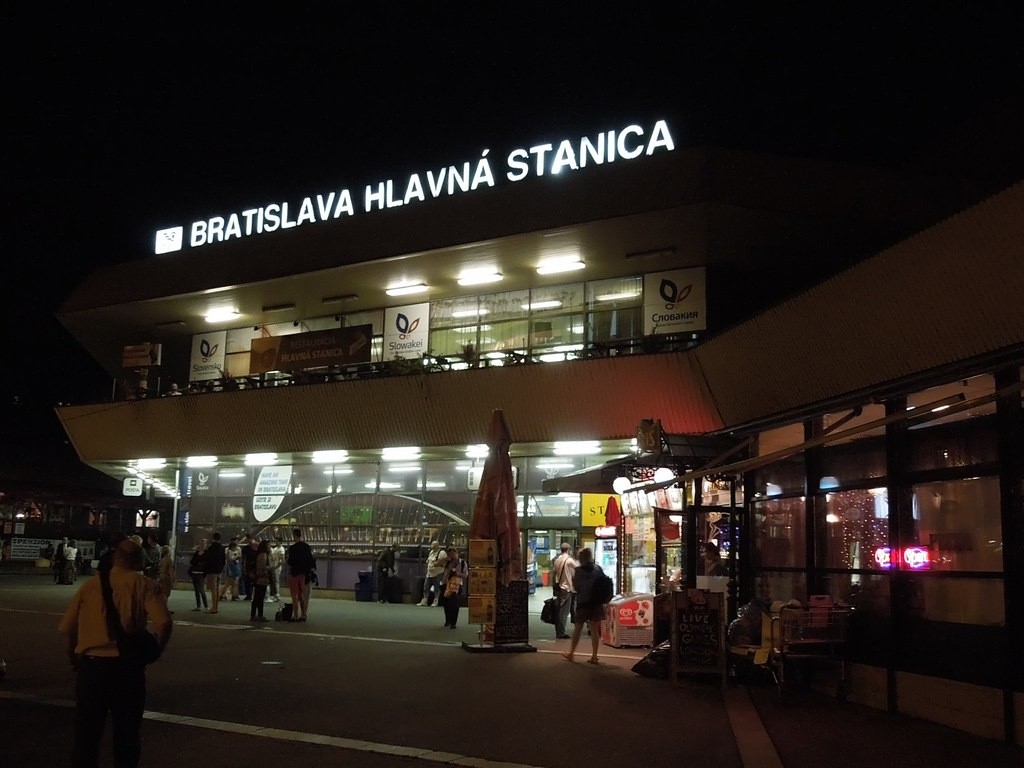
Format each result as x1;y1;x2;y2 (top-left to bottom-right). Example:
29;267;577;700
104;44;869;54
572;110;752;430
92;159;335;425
323;295;359;305
626;248;675;262
156;320;185;330
253;325;270;338
335;315;351;326
262;305;296;314
293;321;310;334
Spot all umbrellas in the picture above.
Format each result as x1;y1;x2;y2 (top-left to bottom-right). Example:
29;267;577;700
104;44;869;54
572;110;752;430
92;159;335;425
472;408;521;646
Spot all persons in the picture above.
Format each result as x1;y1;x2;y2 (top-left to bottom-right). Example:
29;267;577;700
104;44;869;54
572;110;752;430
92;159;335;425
703;542;727;576
54;537;78;582
219;537;242;602
197;533;226;613
144;533;174;598
416;541;448;607
442;549;468;629
287;529;313;622
242;534;285;621
554;543;581;638
170;383;182;395
377;543;399;603
56;541;173;768
188;539;209;611
561;548;607;664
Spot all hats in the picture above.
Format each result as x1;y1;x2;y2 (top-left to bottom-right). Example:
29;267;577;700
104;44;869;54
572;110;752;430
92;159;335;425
127;535;144;549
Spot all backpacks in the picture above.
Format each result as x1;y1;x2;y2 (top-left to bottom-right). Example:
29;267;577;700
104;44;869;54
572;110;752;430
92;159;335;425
245;550;269;581
587;565;615;605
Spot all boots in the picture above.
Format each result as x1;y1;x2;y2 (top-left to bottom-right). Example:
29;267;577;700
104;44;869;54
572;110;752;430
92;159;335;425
431;598;439;607
416;597;427;606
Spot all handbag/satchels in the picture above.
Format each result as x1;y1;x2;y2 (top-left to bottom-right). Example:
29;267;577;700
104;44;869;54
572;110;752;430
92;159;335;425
443;575;463;599
552;583;560;596
117;630;155;664
541;598;560;625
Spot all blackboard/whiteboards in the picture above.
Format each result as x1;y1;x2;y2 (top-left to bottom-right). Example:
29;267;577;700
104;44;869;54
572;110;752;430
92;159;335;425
494;580;529;644
671;588;726;674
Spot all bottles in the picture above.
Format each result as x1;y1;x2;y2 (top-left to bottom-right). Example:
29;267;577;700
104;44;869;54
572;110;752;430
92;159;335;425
486;599;493;623
487;542;494;566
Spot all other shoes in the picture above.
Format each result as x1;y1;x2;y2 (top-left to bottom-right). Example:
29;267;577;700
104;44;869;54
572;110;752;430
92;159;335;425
561;652;574;660
204;608;209;613
587;656;598;665
192;607;201;611
288;616;307;623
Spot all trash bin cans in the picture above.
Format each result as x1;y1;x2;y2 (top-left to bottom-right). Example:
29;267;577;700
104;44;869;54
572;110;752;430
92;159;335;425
415;576;435;606
542;573;549;586
355;571;373;602
388;576;403;603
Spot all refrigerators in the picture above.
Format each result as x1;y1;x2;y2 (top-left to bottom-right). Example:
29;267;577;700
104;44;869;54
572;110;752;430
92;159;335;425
601;593;655;648
